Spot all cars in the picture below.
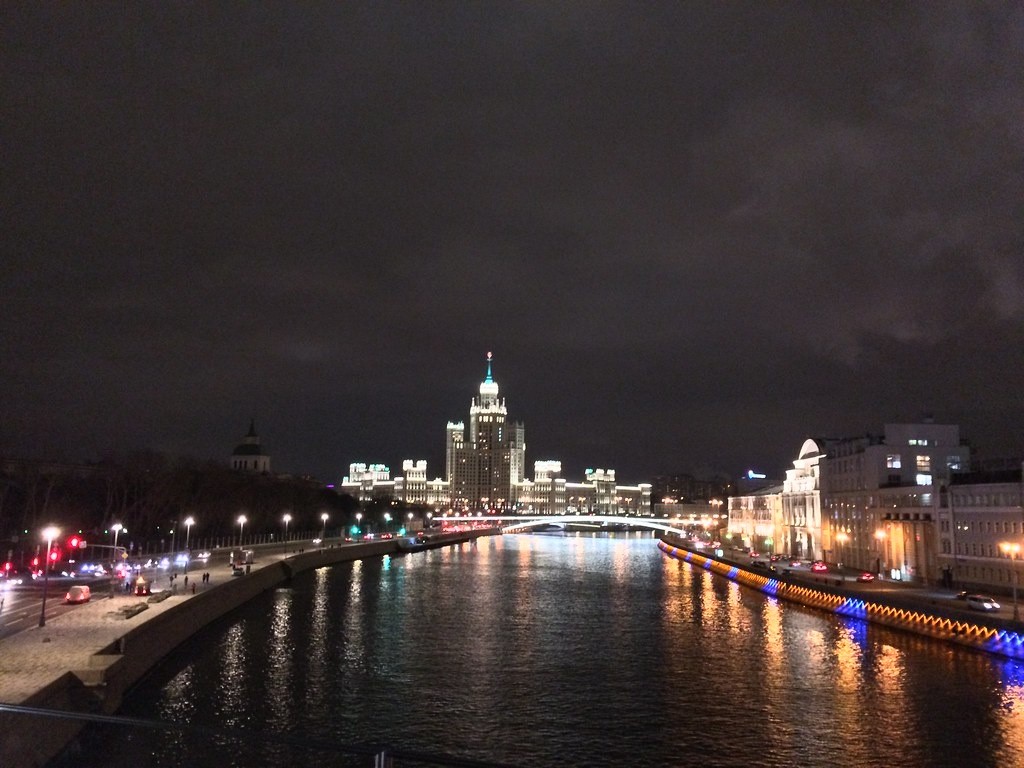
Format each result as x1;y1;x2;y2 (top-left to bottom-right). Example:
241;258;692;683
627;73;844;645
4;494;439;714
65;585;91;605
966;595;1002;613
81;552;212;581
312;521;505;548
811;560;828;572
134;580;151;596
689;531;800;568
858;572;874;583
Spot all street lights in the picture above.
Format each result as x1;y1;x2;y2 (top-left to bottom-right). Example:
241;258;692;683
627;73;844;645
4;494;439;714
282;514;292;552
383;513;391;533
1002;542;1023;623
836;534;850;580
875;528;890;572
321;513;330;547
236;514;247;545
184;516;195;549
109;523;124;597
355;512;362;542
407;513;414;532
38;527;61;627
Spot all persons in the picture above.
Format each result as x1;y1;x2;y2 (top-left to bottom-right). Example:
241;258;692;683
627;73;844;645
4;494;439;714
125;583;129;592
202;573;210;584
191;583;196;595
184;576;188;586
169;576;173;587
298;548;304;554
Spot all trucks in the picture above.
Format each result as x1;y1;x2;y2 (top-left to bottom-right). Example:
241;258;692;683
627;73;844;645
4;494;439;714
227;548;255;564
